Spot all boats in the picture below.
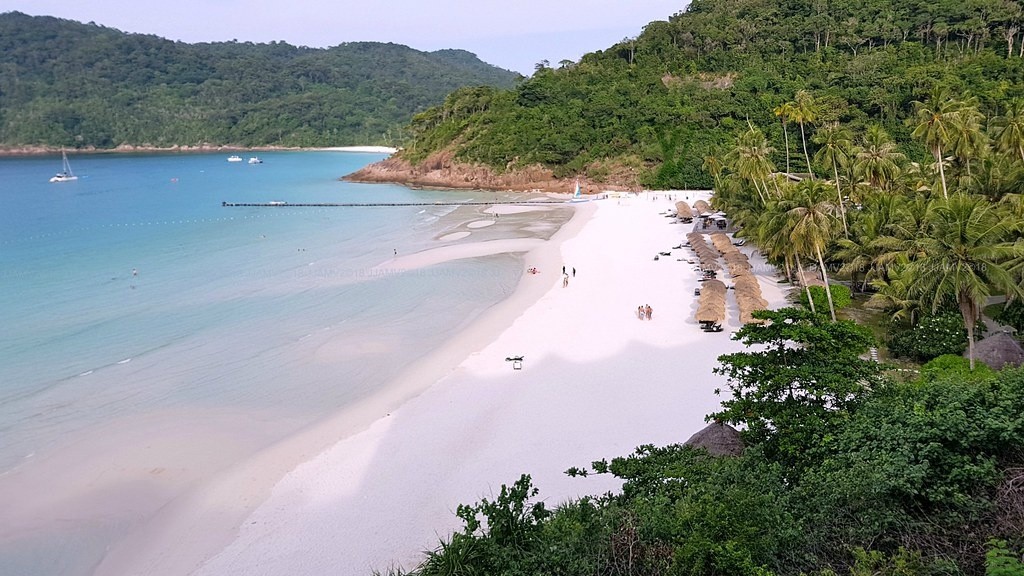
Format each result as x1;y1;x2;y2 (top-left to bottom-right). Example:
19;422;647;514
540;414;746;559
227;155;243;162
248;157;264;164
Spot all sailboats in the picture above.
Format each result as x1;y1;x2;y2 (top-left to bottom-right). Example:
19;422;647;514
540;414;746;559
48;144;78;182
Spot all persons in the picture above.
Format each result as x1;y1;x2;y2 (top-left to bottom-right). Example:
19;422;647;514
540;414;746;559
526;267;540;274
637;304;653;320
654;240;716;281
561;265;576;288
